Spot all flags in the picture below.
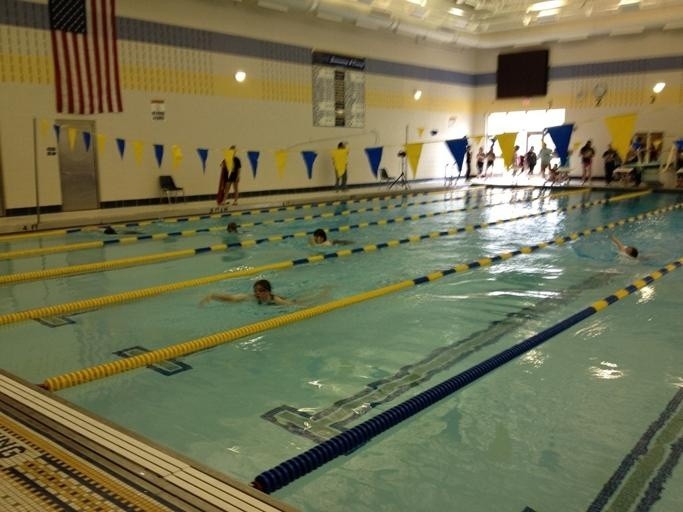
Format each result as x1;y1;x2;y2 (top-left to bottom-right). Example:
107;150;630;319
44;1;123;117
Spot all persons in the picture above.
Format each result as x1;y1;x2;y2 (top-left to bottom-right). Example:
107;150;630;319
304;228;350;247
610;233;638;259
464;136;644;187
218;145;240;206
331;142;348;190
197;279;343;309
224;222;239;234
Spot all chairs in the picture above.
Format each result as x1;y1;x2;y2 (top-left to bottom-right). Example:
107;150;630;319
378;169;397;191
158;175;185;207
